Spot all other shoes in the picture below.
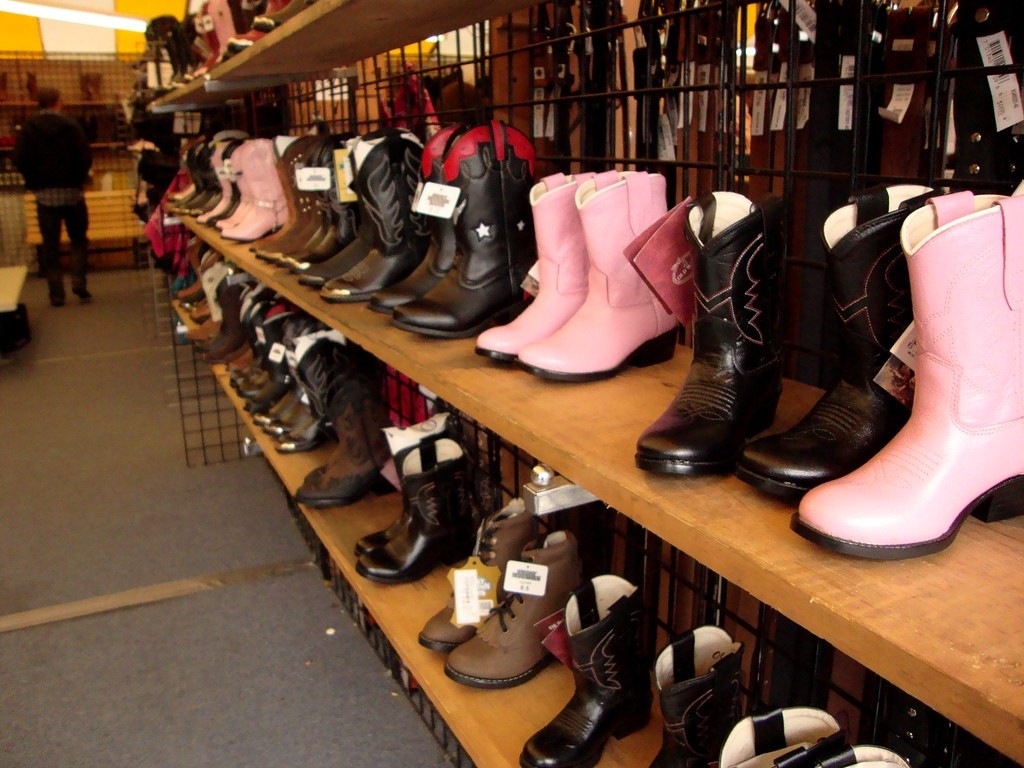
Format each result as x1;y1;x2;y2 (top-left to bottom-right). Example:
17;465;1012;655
72;286;92;299
51;297;64;306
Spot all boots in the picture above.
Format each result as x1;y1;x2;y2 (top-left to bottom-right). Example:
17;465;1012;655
132;1;1024;768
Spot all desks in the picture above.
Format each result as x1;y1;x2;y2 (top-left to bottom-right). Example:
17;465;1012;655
0;265;31;358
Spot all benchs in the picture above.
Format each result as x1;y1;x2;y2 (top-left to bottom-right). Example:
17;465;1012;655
23;189;147;272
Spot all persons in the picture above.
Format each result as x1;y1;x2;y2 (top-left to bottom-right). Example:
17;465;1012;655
10;88;94;307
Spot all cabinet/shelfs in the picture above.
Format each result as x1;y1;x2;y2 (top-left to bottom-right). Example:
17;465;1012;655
159;0;1024;768
